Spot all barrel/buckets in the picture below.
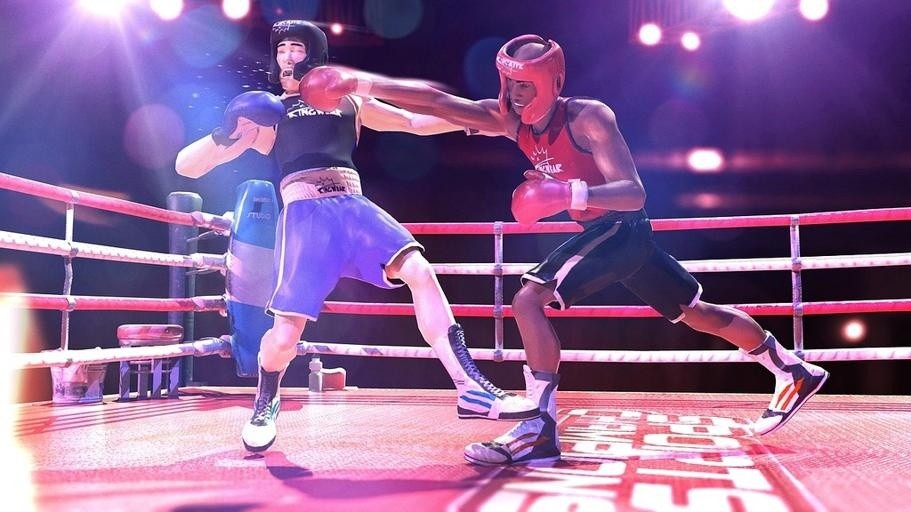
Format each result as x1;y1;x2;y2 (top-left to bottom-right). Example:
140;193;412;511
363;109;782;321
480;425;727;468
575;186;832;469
50;363;107;402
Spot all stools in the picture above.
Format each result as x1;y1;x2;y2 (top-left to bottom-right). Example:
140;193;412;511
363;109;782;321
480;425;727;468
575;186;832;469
113;324;182;401
